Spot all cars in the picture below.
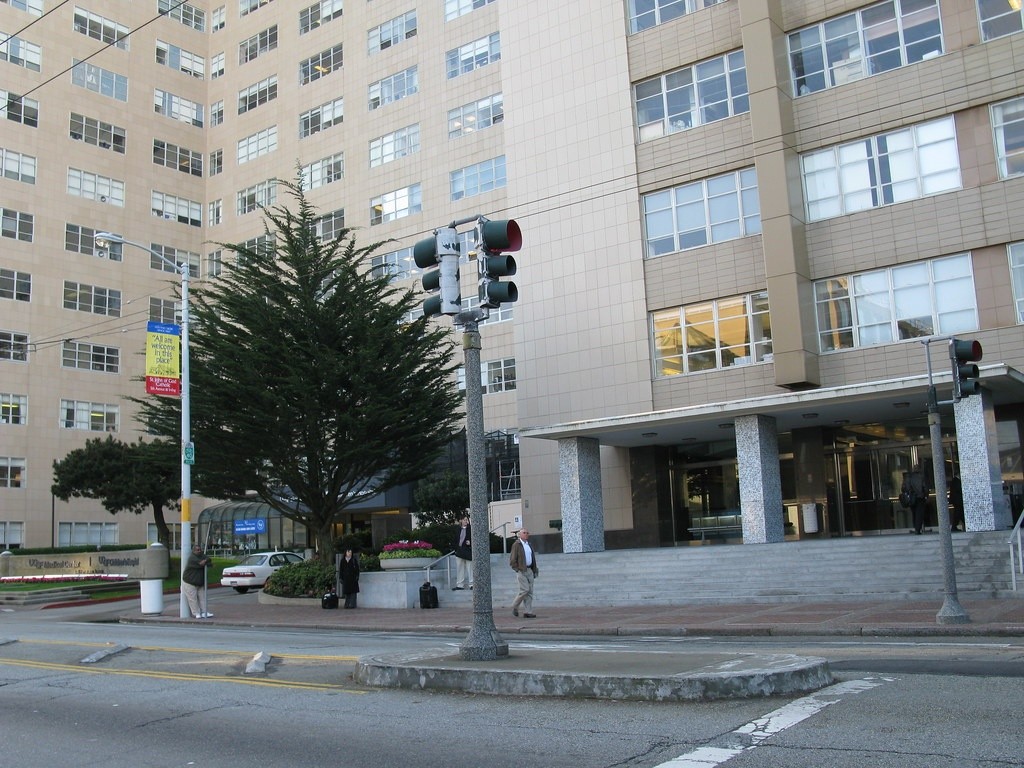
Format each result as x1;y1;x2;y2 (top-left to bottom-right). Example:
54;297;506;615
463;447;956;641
220;551;307;594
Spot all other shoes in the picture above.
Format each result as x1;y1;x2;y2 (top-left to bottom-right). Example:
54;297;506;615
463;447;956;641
470;586;473;590
512;607;518;616
913;529;921;534
523;613;537;618
451;587;463;591
950;526;961;532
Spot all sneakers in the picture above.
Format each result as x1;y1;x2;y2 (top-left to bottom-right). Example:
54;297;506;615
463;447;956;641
201;612;213;617
196;614;202;619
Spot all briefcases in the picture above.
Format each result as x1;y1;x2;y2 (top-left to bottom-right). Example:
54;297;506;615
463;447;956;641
419;586;438;609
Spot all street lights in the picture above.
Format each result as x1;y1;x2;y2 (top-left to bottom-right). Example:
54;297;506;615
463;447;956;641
93;231;194;618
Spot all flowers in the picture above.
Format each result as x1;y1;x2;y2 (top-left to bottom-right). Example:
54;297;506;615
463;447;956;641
377;540;442;559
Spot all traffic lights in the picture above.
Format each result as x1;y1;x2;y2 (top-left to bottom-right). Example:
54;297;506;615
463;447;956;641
948;338;983;398
474;216;523;310
413;227;461;319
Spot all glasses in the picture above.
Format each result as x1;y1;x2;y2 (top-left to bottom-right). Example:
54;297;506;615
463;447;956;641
521;532;529;534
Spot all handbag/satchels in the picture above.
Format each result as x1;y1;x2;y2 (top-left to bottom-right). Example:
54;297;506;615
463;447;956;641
322;592;338;609
899;492;911;507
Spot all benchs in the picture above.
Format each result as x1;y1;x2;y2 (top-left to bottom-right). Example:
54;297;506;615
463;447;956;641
686;516;743;545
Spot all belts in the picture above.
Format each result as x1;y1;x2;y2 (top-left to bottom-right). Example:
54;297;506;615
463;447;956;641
526;566;531;568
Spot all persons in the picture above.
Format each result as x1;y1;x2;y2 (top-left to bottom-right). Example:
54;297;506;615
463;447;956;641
451;514;472;591
899;464;929;534
339;545;359;609
949;471;965;532
183;545;214;619
510;529;537;618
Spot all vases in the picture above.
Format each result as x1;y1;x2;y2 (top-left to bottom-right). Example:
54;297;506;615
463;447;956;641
380;558;440;571
